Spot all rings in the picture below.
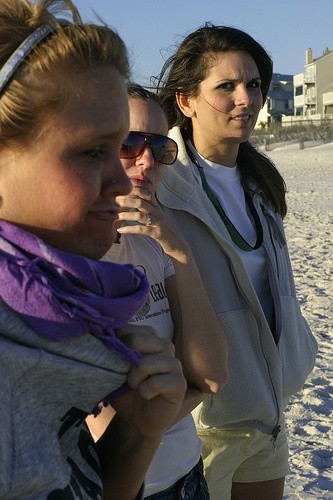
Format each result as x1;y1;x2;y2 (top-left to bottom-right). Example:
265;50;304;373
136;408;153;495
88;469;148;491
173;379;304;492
146;213;153;227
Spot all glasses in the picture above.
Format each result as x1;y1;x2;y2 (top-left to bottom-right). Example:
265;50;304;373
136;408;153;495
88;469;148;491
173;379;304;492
119;131;179;165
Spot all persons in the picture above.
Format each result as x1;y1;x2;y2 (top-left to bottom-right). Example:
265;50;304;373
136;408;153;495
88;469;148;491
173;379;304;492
0;0;186;500
150;24;319;499
86;83;229;500
